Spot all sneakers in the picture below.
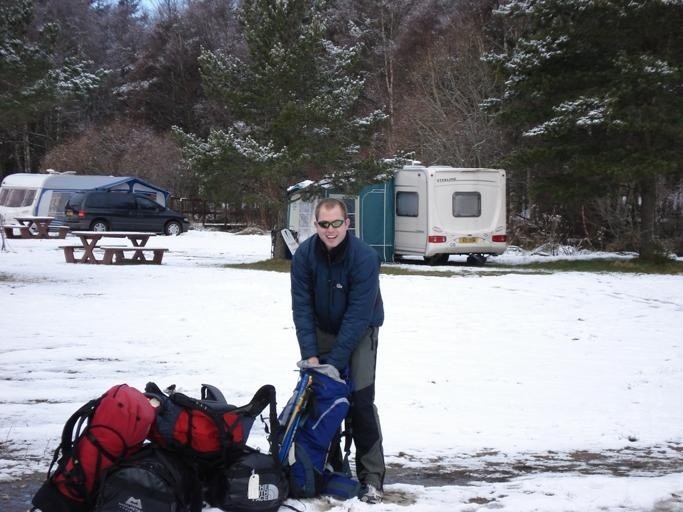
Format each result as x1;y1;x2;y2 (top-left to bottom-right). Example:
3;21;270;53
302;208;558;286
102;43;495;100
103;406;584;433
361;482;383;505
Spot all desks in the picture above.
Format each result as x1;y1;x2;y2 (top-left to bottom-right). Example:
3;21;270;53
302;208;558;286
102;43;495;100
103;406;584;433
72;230;156;263
14;216;54;238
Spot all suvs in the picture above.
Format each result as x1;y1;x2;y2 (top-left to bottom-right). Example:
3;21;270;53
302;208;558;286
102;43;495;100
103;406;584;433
62;191;191;238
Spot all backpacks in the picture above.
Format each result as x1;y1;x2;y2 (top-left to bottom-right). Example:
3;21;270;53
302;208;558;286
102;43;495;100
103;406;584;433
267;360;363;498
30;382;288;512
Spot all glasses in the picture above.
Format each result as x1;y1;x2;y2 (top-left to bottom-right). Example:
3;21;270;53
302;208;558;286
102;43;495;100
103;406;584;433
318;220;343;228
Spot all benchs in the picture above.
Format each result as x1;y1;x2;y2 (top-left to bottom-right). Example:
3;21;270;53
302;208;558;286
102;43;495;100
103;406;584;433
58;245;103;262
41;223;70;240
0;224;31;239
97;245;168;264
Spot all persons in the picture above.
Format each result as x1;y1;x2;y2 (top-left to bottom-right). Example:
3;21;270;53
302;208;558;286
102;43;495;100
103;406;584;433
291;198;385;503
